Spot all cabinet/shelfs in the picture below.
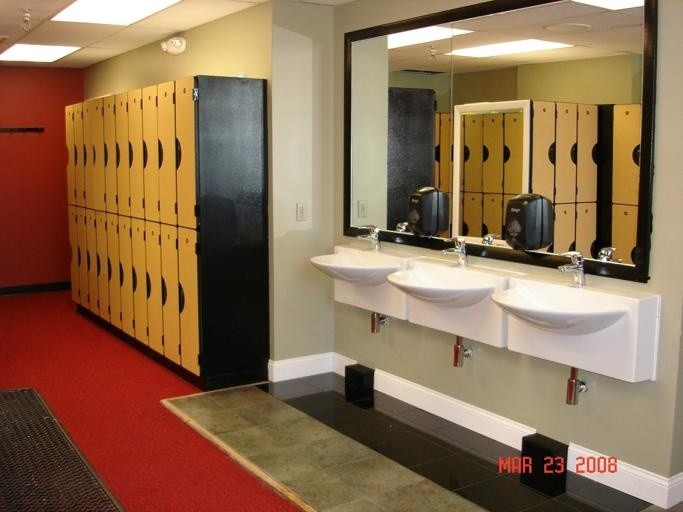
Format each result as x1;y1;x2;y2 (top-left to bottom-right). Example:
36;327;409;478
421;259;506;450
62;75;269;391
429;99;642;268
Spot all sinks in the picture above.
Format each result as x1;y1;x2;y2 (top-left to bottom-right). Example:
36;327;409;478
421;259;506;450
390;262;493;309
493;276;625;333
310;252;400;287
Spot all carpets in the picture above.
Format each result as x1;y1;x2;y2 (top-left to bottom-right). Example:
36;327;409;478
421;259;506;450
160;385;490;511
1;385;124;510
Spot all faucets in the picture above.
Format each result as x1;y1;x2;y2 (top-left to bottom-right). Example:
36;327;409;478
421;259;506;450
558;253;584;286
395;222;409;231
481;235;495;247
441;235;468;266
357;225;380;250
596;247;624;263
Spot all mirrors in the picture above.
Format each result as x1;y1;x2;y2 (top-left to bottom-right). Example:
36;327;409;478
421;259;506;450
343;0;658;282
